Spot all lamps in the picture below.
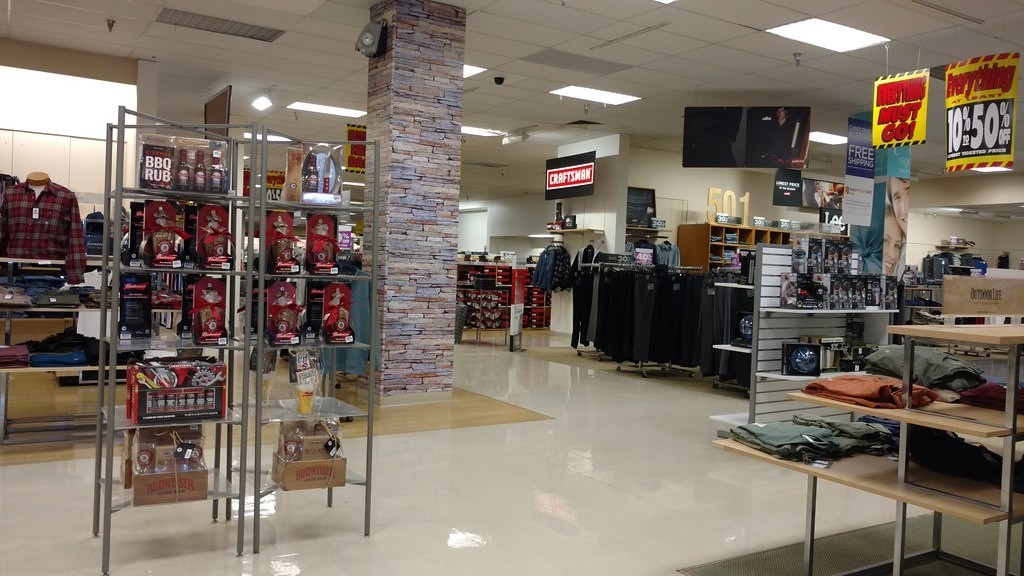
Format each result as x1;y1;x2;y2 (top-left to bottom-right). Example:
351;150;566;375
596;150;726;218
501;128;532;146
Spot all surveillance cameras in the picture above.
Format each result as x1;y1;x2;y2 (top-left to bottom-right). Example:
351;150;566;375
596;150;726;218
494;77;505;85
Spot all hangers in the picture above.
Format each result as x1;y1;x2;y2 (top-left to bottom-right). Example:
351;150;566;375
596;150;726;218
639;233;648;243
663;237;671;244
586;240;594;249
582;264;736;278
547;240;563;251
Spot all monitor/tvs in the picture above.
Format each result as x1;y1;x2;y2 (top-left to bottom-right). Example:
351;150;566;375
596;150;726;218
682;106;812;169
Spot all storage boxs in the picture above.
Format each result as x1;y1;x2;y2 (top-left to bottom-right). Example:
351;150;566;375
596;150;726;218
118;448;133;490
131;360;227;424
131;460;209;507
125;355;216;419
271;450;347;492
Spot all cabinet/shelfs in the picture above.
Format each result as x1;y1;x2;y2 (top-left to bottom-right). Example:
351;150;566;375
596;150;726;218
457;263;551;330
677;222;1024;576
0;101;381;576
